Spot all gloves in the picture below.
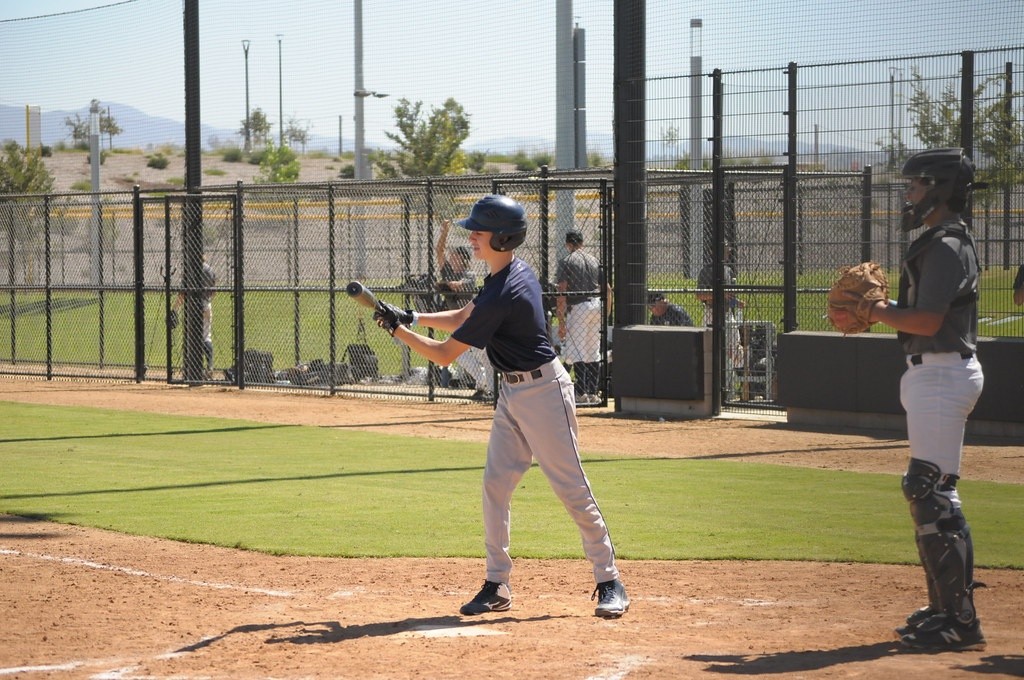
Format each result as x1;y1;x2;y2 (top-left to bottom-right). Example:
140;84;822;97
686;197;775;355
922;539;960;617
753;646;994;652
373;301;414;336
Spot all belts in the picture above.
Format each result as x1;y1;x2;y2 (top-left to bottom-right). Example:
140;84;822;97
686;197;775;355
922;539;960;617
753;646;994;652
500;369;542;385
911;351;973;366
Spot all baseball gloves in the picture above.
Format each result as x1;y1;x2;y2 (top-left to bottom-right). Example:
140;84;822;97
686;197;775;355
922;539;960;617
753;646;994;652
165;309;179;329
826;260;890;339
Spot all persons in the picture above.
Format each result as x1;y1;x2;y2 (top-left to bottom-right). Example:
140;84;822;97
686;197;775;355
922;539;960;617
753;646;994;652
648;292;693;326
827;147;985;649
697;237;746;400
556;229;613;404
373;195;631;619
436;218;495;398
166;248;217;380
1013;264;1024;305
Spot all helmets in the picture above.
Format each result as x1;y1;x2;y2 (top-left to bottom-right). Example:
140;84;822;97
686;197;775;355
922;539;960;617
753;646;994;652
900;149;976;232
456;195;527;252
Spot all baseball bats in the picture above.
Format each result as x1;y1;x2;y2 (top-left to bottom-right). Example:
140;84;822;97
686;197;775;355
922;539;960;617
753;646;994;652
346;280;385;314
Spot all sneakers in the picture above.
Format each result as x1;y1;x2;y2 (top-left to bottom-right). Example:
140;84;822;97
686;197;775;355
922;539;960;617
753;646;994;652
901;618;986;651
591;579;630;616
460;579;512;615
893;604;936;640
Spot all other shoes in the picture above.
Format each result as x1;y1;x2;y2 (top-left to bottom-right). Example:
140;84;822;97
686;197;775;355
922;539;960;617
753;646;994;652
575;393;589;406
589;394;601;403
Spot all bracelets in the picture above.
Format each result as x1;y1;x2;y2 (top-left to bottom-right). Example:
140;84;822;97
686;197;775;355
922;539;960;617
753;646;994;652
409;311;418;326
